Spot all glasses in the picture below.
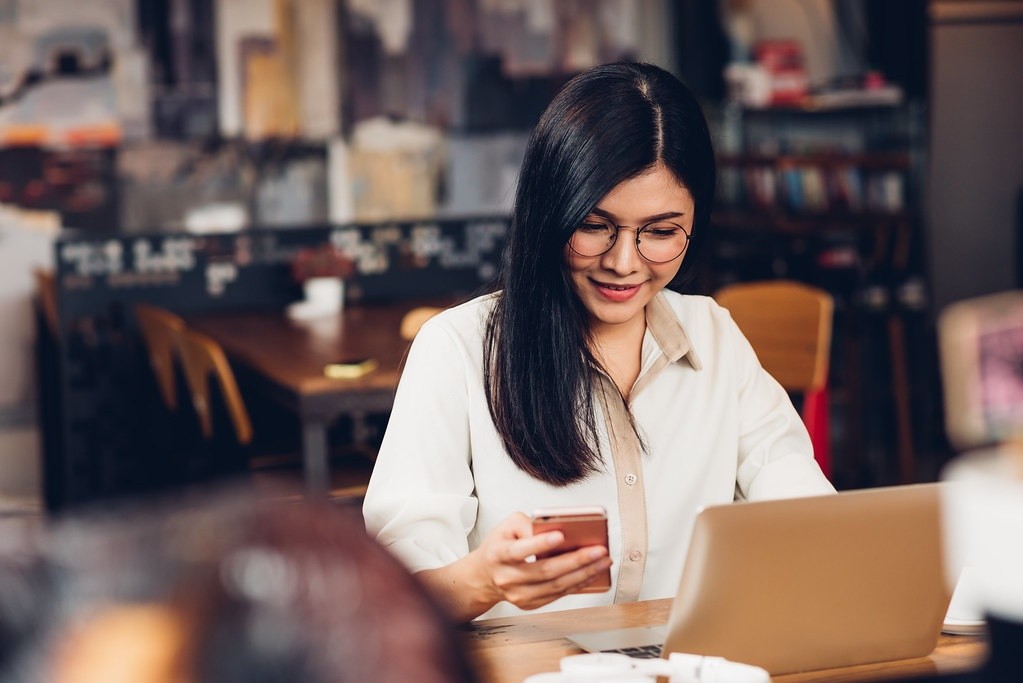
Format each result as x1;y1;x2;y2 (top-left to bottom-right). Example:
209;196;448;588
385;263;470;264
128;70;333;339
559;215;690;263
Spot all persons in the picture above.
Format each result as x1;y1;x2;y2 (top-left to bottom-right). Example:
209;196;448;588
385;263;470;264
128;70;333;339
363;64;841;629
0;479;497;682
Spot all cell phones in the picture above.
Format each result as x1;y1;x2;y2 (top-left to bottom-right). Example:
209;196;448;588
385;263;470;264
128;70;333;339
532;506;612;594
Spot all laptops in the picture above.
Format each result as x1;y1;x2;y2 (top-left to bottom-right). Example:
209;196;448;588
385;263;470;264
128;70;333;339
565;481;964;673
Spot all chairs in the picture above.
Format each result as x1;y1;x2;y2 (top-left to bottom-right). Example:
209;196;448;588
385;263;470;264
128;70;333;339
133;305;261;451
939;292;1023;445
718;283;831;475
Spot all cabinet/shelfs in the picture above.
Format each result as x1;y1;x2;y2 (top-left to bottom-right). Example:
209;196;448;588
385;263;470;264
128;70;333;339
707;100;932;320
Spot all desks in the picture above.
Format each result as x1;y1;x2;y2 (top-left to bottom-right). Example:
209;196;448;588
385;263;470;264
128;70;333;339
187;307;453;503
466;597;986;683
